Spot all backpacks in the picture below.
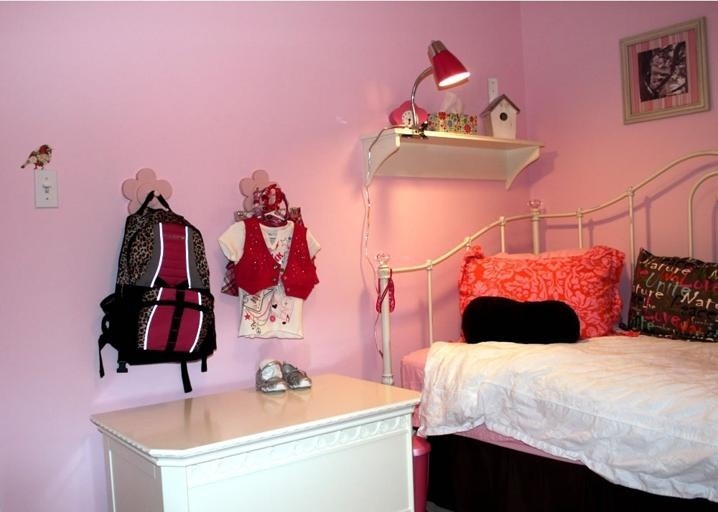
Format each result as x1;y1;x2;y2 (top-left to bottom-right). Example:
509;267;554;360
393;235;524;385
97;191;216;363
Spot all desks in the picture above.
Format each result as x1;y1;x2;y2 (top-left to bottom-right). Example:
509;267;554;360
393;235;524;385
89;374;422;512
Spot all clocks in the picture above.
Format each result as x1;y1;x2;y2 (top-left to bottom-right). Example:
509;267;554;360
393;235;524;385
389;100;428;126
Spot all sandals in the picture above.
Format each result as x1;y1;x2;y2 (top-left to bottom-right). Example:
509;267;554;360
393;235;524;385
256;361;287;394
282;363;311;389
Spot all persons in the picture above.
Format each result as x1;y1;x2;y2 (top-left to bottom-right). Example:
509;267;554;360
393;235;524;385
644;51;687;93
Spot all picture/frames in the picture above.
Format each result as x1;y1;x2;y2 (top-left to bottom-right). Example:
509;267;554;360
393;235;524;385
618;16;710;125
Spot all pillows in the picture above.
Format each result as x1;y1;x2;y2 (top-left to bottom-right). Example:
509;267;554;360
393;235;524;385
446;244;718;344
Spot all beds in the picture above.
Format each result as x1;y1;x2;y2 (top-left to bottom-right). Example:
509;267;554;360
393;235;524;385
377;151;718;503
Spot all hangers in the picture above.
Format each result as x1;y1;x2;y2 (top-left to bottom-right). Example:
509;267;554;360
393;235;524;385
249;206;285;222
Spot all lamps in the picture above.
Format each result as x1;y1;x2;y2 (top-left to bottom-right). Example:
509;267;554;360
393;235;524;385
411;40;471;126
